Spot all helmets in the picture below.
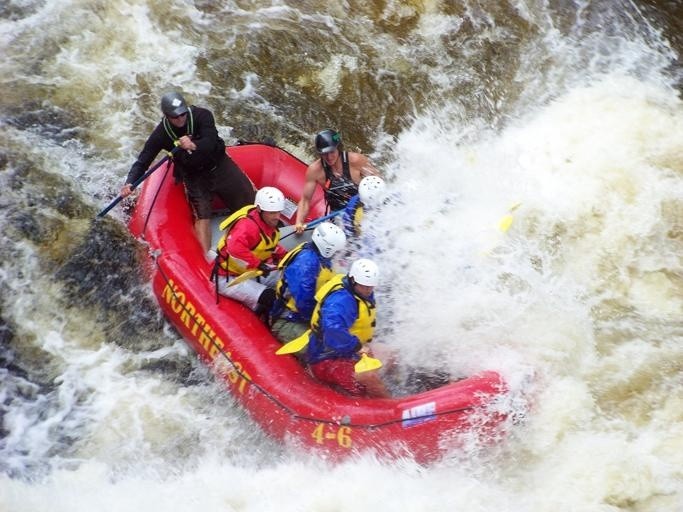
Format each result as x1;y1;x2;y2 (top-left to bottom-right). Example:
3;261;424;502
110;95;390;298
315;129;341;154
311;221;346;258
359;176;387;207
161;92;188;117
254;186;285;212
348;258;380;286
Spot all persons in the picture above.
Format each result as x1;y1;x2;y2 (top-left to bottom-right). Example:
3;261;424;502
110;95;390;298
120;93;257;252
214;130;398;399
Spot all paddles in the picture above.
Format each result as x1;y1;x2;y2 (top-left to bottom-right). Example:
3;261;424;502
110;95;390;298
354;353;382;374
226;268;279;287
275;329;312;355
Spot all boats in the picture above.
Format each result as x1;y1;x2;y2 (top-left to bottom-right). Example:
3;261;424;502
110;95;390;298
125;144;554;469
